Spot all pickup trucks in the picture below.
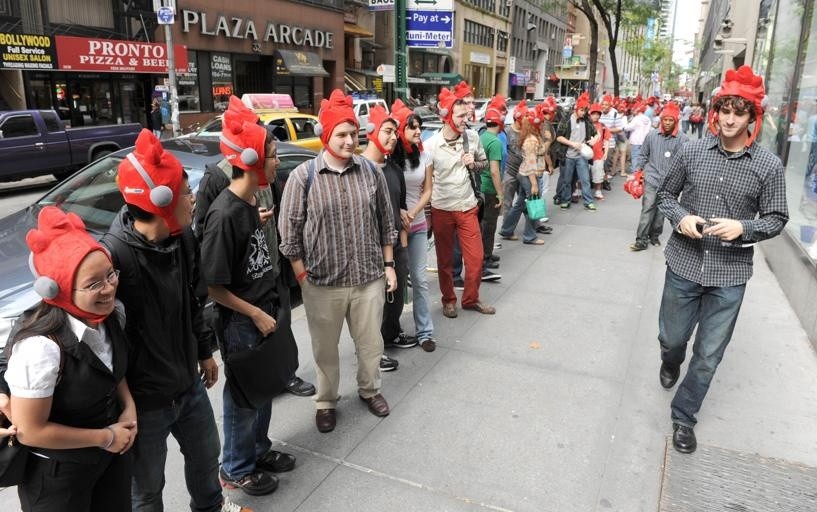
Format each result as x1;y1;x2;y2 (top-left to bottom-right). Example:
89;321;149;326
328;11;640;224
0;109;146;186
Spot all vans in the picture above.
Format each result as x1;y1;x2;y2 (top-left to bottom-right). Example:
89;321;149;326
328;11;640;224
347;97;390;144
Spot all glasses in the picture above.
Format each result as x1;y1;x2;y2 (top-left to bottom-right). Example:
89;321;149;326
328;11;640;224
74;267;119;297
183;187;194;199
266;153;278;162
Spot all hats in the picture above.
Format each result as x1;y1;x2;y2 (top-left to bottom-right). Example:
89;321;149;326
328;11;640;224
117;128;184;214
219;119;267;173
27;204;110;308
313;89;414;143
576;91;681;120
225;94;261;126
437;80;557;128
713;65;765;114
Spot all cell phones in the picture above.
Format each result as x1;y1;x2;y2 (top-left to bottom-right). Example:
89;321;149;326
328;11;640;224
697;224;708;235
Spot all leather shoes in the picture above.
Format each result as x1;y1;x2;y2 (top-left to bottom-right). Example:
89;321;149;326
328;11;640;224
473;303;496;314
660;362;680;389
315;408;336;432
648;235;660;247
289;376;315;396
442;302;457;318
360;394;390;416
630;240;648;249
672;422;696;454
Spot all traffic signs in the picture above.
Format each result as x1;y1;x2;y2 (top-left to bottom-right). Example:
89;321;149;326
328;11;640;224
404;0;455;9
403;7;454;49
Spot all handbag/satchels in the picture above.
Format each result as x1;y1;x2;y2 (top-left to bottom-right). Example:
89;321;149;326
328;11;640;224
0;335;65;489
223;310;300;410
689;113;703;123
545;141;559;171
476;188;486;222
525;194;546;221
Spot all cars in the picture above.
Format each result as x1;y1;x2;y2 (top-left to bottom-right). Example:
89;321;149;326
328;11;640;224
173;93;230;112
1;135;322;395
417;93;576;146
196;92;363;160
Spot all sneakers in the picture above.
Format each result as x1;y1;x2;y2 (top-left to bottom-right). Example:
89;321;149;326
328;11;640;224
219;449;295;512
499;217;553;245
554;174;613;210
454;254;501;290
380;332;435;372
